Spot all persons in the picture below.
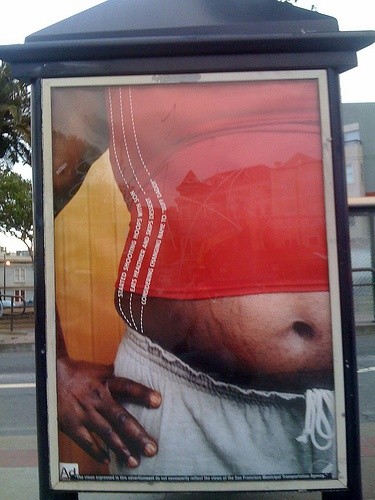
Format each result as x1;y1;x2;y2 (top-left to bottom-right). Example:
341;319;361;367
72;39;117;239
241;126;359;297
52;78;340;480
